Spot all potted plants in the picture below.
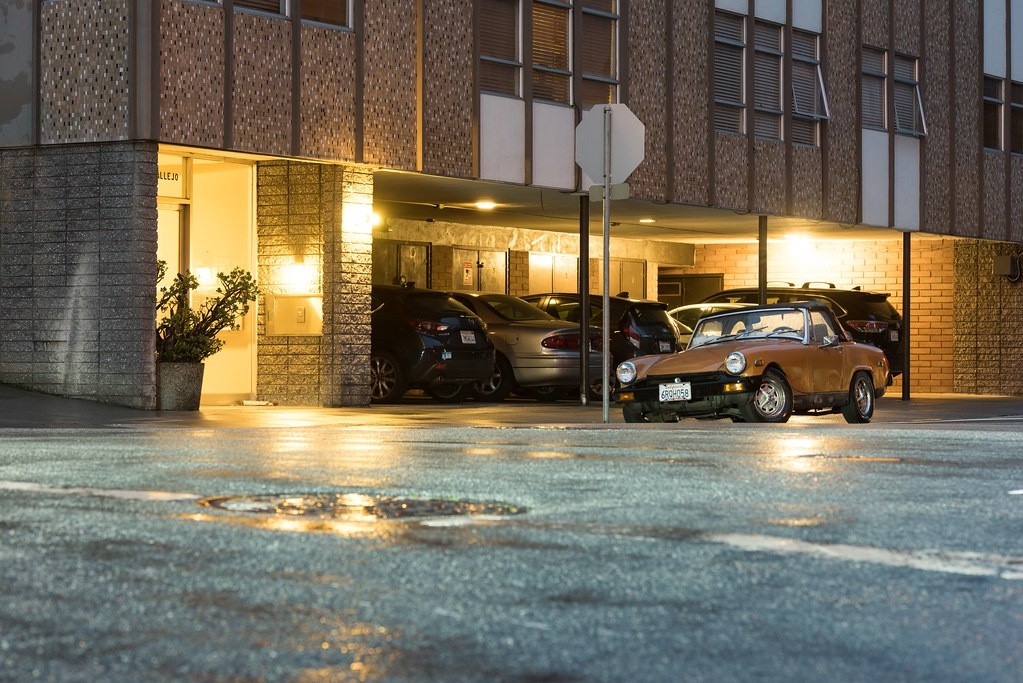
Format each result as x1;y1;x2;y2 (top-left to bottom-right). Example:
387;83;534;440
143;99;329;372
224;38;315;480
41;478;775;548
155;260;261;411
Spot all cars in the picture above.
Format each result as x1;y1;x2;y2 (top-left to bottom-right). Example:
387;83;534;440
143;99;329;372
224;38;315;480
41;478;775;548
437;290;614;403
557;302;694;351
371;283;497;405
614;301;893;423
493;291;684;401
667;303;836;337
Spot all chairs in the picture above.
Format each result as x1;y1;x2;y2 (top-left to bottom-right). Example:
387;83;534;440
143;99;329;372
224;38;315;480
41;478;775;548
801;324;829;342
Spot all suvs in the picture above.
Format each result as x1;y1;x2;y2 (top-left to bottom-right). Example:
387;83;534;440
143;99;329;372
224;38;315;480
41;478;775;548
675;281;906;399
